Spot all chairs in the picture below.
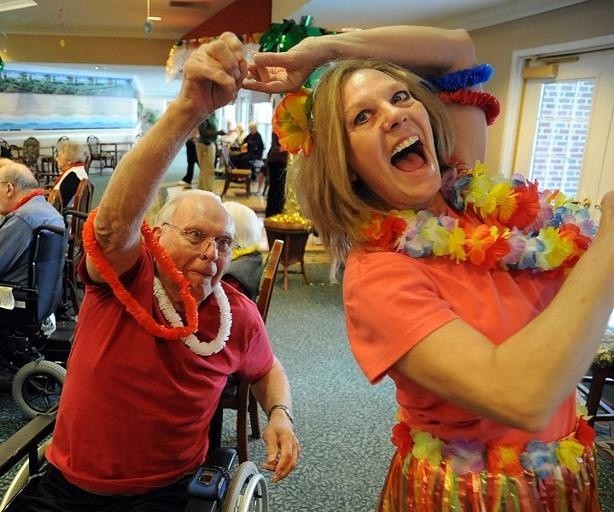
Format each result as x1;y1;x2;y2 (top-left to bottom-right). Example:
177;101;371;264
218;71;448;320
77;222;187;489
210;239;285;464
0;135;117;184
65;179;95;315
220;146;253;200
579;338;614;429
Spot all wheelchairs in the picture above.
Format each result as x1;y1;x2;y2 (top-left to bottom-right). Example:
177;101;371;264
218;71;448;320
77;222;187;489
0;403;269;512
0;208;91;418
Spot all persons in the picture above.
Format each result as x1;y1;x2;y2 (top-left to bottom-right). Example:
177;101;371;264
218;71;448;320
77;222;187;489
0;139;89;370
182;111;288;219
0;32;300;512
234;27;614;512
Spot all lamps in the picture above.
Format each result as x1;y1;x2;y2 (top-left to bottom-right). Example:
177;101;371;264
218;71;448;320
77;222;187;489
144;0;162;34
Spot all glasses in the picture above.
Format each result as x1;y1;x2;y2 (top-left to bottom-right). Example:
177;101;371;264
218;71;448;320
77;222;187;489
162;222;241;256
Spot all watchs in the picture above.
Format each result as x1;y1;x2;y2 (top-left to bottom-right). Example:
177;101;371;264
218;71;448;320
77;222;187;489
266;404;294;424
